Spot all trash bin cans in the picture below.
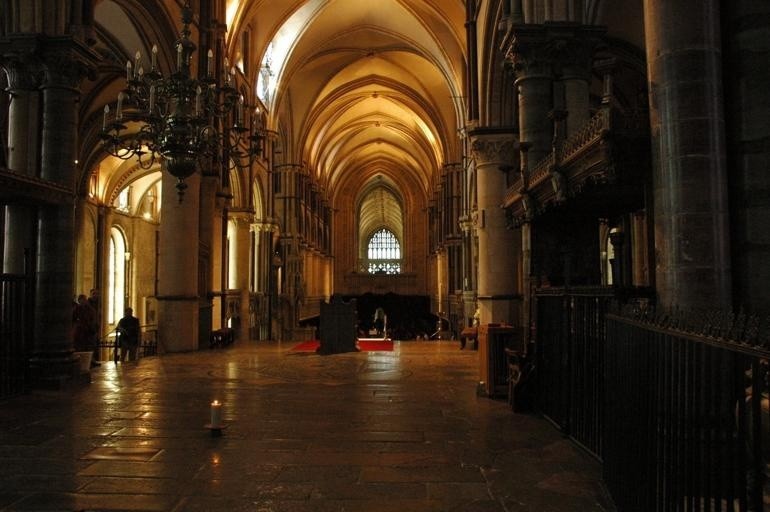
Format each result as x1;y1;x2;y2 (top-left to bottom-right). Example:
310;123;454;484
487;322;518;399
504;343;538;415
74;351;93;372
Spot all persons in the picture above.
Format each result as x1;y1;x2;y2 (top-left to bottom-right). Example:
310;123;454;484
87;289;102;360
117;308;140;362
76;294;97;368
372;306;383;336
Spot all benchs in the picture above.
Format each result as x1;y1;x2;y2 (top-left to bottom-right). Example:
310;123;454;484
460;326;478;351
213;328;235;347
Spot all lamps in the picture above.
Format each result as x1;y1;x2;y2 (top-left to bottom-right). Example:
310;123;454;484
98;0;265;203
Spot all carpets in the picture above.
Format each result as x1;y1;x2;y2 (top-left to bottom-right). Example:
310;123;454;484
288;339;394;351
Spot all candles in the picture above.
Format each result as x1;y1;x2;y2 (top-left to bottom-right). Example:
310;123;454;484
210;404;222;425
